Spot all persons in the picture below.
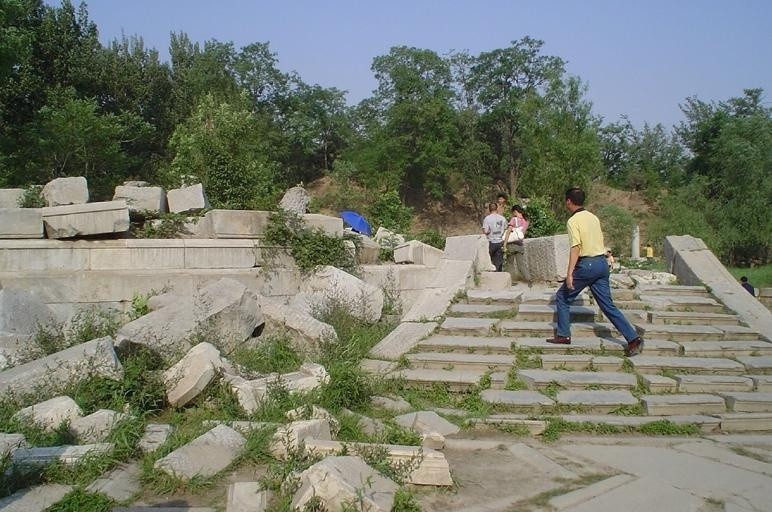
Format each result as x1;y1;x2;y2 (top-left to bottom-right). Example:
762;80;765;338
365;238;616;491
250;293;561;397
606;251;614;267
482;203;508;272
495;193;509;215
501;205;534;287
741;276;754;296
546;188;641;357
646;243;653;260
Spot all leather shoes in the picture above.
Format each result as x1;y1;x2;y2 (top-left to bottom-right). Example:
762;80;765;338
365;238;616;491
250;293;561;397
546;336;571;344
624;336;642;357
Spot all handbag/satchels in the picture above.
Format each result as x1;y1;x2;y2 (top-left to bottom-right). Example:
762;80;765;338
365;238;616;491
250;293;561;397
502;227;524;242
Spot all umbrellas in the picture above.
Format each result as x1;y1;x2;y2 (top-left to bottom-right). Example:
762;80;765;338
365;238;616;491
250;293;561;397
339;210;371;235
